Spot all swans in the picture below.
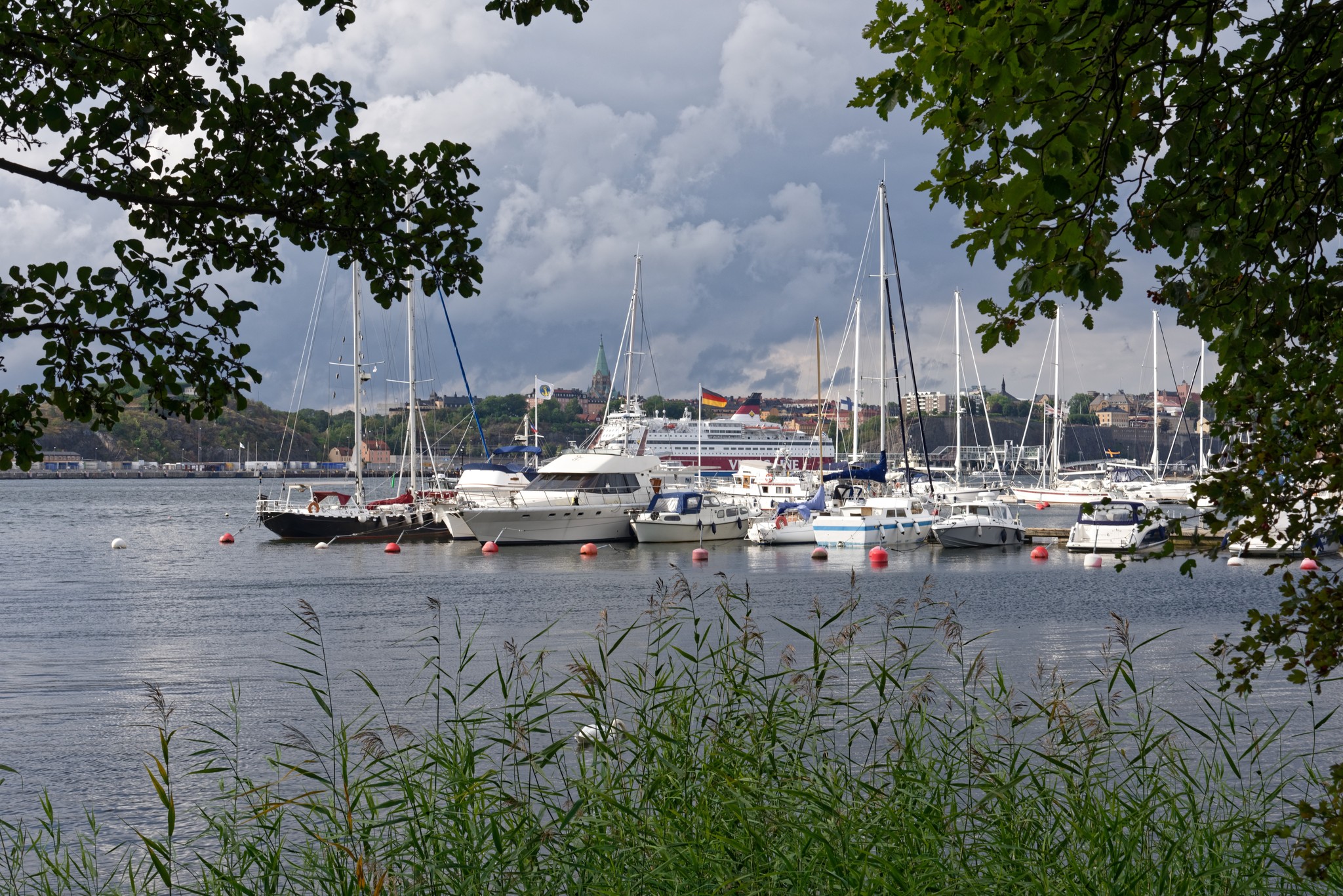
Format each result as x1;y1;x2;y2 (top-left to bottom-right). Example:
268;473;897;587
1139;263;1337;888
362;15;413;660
574;719;627;744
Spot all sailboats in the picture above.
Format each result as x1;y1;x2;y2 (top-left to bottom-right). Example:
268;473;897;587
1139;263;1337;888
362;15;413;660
239;182;1338;564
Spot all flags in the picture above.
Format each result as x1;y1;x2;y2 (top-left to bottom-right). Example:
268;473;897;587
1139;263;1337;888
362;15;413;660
1103;447;1121;458
530;424;538;433
536;377;555;400
240;442;245;449
700;385;727;408
